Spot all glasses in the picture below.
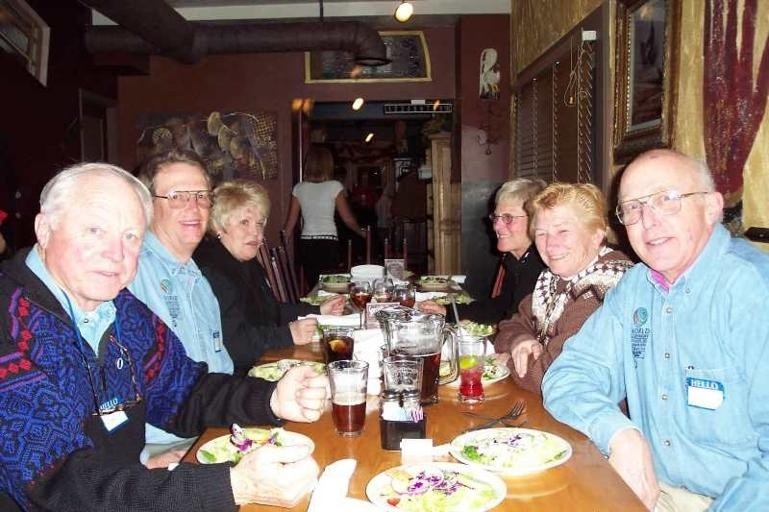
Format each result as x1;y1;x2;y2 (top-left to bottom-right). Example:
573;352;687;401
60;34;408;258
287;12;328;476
615;189;710;227
154;189;212;210
488;212;526;225
83;335;144;418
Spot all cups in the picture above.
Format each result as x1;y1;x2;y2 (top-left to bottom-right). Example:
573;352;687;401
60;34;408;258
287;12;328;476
399;438;435;480
275;254;526;450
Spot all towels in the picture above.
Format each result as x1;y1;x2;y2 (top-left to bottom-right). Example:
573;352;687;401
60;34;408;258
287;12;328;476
307;458;373;512
296;312;363;326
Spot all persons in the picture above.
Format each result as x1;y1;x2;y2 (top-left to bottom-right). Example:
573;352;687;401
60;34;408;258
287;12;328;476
285;147;367;292
420;177;548;329
540;149;769;512
0;162;329;512
126;148;234;469
494;181;635;418
192;178;346;379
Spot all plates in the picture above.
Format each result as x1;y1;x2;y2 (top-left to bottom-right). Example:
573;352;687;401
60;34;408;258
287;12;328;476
447;428;576;477
502;474;575;502
363;461;508;512
194;428;318;467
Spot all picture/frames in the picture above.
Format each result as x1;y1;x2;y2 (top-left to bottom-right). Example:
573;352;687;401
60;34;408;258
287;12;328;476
305;30;432;84
614;0;675;144
